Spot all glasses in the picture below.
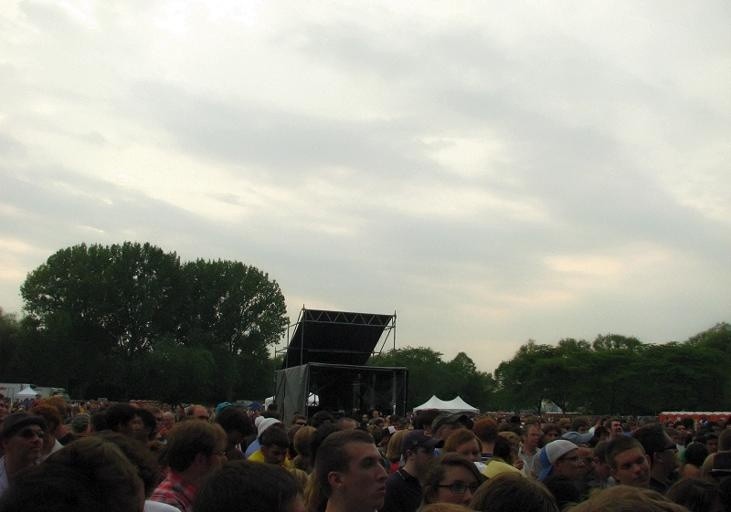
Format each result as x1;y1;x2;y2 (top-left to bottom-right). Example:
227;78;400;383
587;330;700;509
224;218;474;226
556;455;579;462
17;428;45;438
437;480;483;496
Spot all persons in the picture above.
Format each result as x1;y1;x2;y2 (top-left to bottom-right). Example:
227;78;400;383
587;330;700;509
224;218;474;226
0;394;730;512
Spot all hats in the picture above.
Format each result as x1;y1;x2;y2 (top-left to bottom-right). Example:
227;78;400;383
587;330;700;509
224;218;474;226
254;415;281;438
431;413;468;434
532;439;580;481
561;426;595;444
0;411;49;441
402;429;446;451
215;401;232;416
188;404;210;419
